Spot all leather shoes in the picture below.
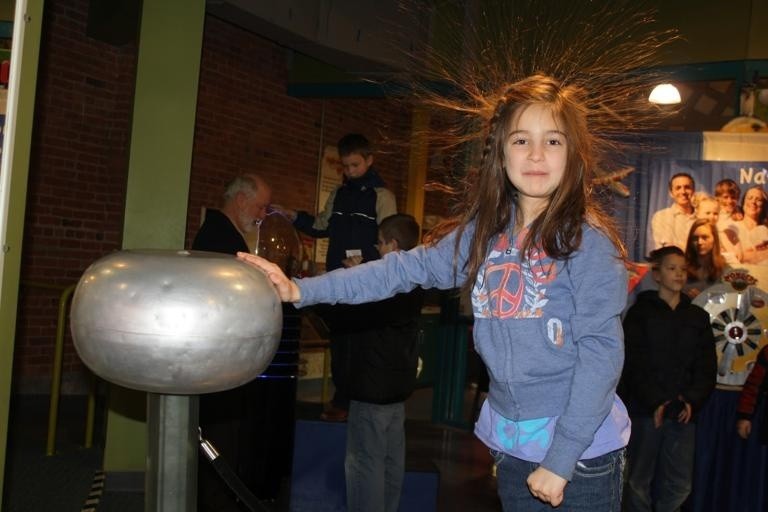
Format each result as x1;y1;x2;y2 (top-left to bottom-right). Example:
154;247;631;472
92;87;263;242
320;407;348;422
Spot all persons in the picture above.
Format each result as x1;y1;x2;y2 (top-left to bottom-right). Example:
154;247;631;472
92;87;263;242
615;173;767;512
235;2;682;511
191;132;422;511
